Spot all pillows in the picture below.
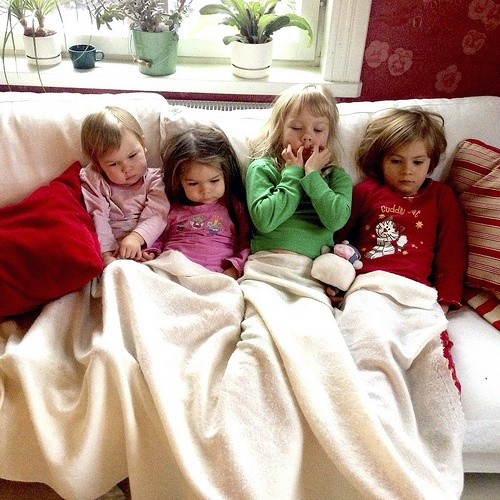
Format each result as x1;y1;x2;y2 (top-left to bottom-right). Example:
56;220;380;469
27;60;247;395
0;160;103;319
444;139;500;331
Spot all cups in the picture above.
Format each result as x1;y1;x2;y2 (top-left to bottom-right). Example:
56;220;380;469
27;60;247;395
68;45;104;69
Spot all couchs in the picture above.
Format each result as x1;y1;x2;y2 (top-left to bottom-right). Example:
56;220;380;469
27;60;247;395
0;93;500;476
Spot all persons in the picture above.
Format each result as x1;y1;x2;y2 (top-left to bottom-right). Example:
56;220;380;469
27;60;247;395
324;105;469;317
137;122;251;280
77;104;171;271
246;82;352;262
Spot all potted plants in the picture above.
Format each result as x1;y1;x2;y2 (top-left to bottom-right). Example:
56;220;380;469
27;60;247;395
199;0;313;80
76;0;194;76
0;0;66;93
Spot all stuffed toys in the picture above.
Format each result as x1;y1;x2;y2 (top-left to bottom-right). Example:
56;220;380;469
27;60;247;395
309;240;363;294
